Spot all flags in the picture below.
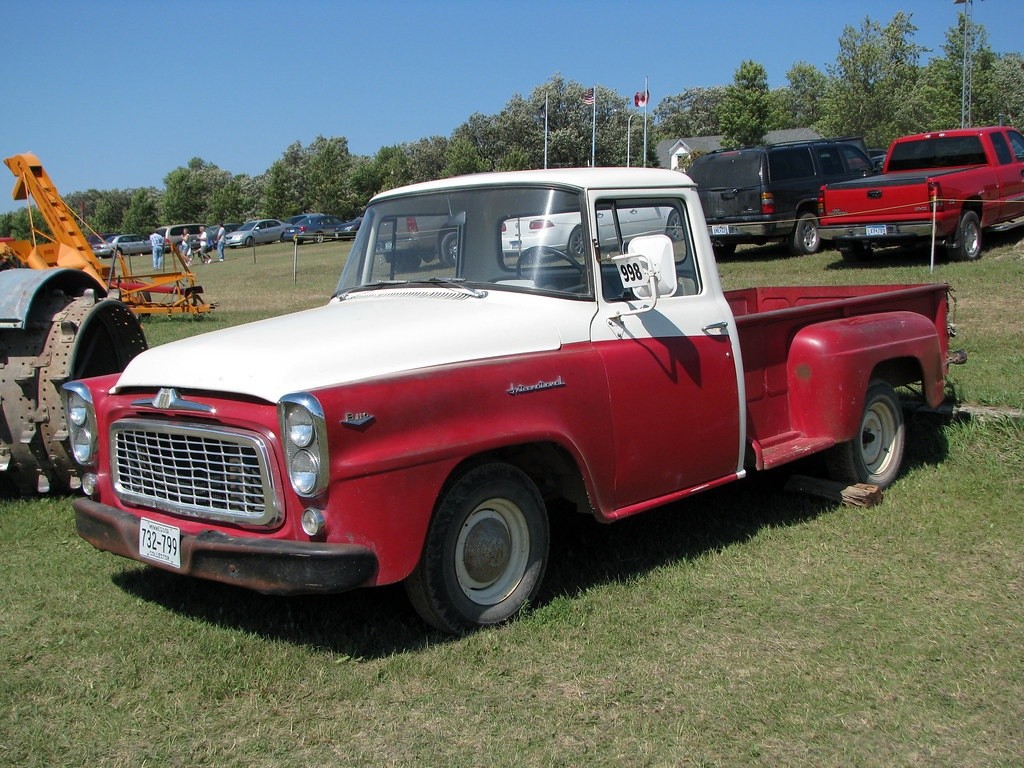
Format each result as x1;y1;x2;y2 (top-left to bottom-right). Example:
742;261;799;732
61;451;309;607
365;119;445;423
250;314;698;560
539;101;546;121
580;88;595;105
635;90;650;107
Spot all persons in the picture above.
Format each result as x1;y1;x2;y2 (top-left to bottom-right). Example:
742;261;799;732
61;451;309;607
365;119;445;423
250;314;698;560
180;228;193;267
194;226;214;264
216;221;227;262
150;229;165;271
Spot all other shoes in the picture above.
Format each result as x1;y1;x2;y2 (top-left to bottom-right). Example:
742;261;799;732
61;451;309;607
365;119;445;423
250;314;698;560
205;259;211;264
218;258;224;262
186;262;192;267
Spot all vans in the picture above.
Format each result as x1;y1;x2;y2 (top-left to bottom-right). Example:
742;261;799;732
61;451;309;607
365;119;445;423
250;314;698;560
151;224;211;252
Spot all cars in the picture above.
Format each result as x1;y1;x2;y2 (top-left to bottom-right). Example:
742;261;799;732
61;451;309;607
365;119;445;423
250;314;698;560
90;234;152;258
192;224;239;248
335;213;365;242
501;206;686;259
287;214;324;223
284;215;349;244
226;219;293;248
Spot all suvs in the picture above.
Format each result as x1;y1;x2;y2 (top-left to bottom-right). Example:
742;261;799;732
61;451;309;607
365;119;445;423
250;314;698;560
688;140;884;256
87;233;116;247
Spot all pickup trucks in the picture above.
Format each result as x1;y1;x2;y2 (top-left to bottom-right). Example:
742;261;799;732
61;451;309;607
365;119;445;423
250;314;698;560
376;213;459;271
58;166;967;638
816;124;1024;261
